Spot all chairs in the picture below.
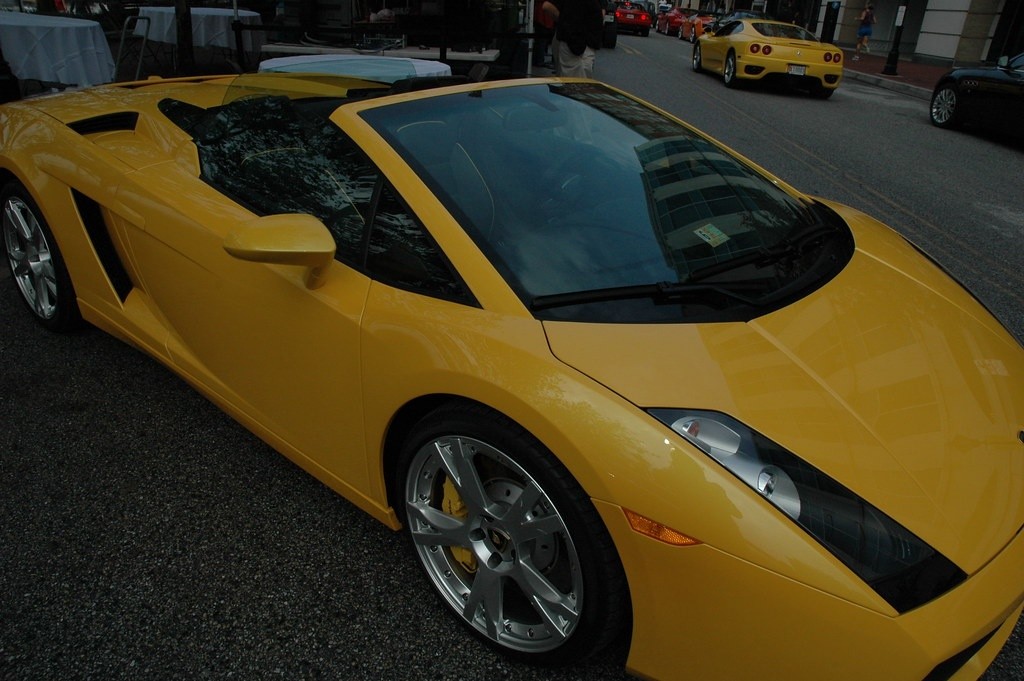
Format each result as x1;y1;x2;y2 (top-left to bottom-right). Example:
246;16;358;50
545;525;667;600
238;121;495;293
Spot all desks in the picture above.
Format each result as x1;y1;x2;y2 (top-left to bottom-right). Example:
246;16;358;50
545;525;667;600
132;7;267;78
0;12;115;100
261;41;500;62
256;55;453;86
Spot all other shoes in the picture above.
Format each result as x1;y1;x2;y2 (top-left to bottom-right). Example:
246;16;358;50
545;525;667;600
866;47;871;54
851;55;859;62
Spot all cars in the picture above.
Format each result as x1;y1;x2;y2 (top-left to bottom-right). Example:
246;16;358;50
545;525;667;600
928;50;1024;146
613;3;654;37
677;12;723;44
655;9;700;36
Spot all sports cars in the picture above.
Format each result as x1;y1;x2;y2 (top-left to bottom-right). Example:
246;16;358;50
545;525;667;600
692;20;846;99
2;67;1023;681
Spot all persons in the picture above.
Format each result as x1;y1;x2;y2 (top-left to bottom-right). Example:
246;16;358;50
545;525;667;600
706;0;716;13
852;0;877;61
534;0;614;81
716;3;726;14
